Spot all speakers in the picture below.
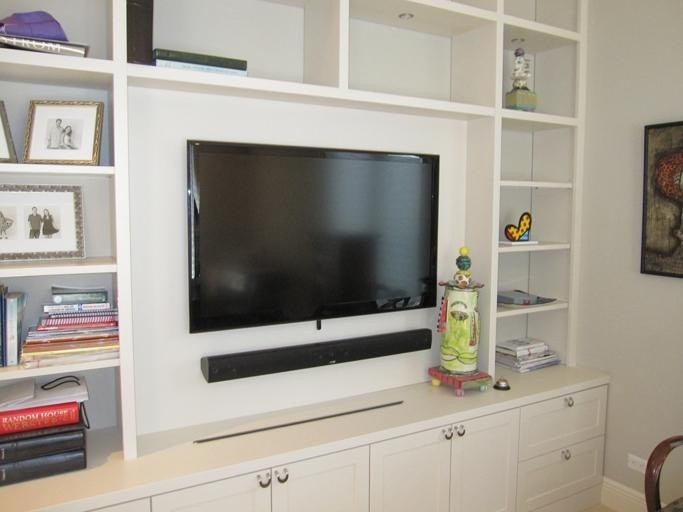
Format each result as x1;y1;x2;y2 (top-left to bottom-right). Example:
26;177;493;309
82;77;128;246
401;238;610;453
201;328;432;383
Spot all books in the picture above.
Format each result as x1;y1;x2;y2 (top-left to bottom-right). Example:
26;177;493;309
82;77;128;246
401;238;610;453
0;283;119;368
154;57;247;78
496;288;557;310
126;0;153;65
495;336;560;373
152;49;248;71
0;376;89;487
0;33;90;58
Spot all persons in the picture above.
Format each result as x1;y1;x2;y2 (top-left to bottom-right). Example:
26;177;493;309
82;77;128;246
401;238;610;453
41;208;58;238
27;206;42;239
46;118;62;149
59;125;76;150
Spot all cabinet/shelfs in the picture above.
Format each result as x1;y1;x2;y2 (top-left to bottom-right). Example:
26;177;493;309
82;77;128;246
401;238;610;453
1;0;591;461
150;446;370;512
370;407;517;512
91;496;152;512
517;383;615;512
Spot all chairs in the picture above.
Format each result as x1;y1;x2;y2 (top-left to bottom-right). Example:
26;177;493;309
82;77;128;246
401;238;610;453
644;435;683;512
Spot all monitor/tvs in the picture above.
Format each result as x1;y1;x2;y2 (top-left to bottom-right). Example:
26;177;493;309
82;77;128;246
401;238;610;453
188;139;439;333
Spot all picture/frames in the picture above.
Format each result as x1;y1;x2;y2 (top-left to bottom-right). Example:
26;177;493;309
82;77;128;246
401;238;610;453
0;184;85;261
640;121;683;279
22;100;105;166
